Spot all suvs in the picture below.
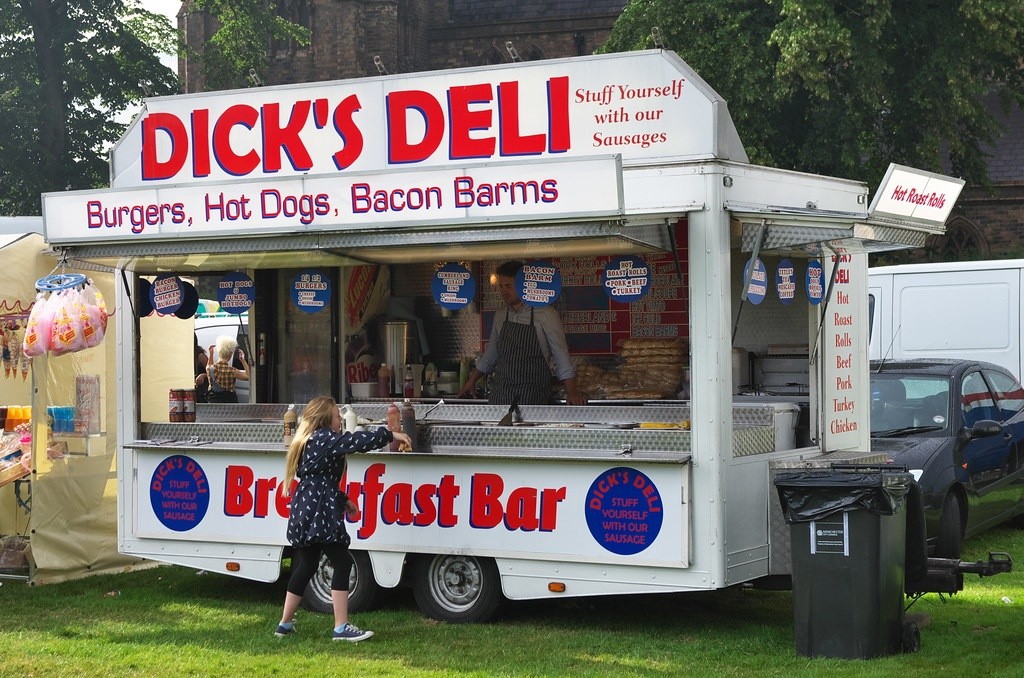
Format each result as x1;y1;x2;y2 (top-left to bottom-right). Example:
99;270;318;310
194;312;249;403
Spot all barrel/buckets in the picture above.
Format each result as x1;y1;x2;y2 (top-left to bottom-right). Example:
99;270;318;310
372;318;417;396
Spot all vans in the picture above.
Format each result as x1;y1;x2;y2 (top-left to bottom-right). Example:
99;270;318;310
868;259;1024;400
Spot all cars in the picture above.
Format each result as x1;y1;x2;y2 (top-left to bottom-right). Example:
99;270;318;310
870;358;1023;561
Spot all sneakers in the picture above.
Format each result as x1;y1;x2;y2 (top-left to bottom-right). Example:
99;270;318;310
331;623;373;642
274;625;296;636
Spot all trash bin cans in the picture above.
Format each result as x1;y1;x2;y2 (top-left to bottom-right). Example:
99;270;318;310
773;461;932;663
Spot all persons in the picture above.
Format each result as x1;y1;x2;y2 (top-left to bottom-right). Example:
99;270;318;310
456;261;588;405
206;339;250;403
194;332;209;403
275;396;411;641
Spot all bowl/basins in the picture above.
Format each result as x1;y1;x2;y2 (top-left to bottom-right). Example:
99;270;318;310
349;381;378;398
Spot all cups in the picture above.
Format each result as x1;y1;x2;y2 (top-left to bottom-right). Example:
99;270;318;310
48;406;75;432
0;404;32;456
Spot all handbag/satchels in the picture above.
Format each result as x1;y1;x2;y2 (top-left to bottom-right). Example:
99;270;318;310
208;391;237;403
22;277;108;359
210;365;222;392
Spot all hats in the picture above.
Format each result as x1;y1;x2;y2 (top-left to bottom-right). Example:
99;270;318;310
919;395;944;409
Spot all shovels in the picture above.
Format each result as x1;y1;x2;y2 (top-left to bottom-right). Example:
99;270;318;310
498;394;521;426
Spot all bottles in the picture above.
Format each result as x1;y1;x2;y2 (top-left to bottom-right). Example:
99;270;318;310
423;359;439;397
402;399;418;452
284;404;298;446
387;401;401;451
338;405;357;436
376;363;389;398
404;364;414;398
